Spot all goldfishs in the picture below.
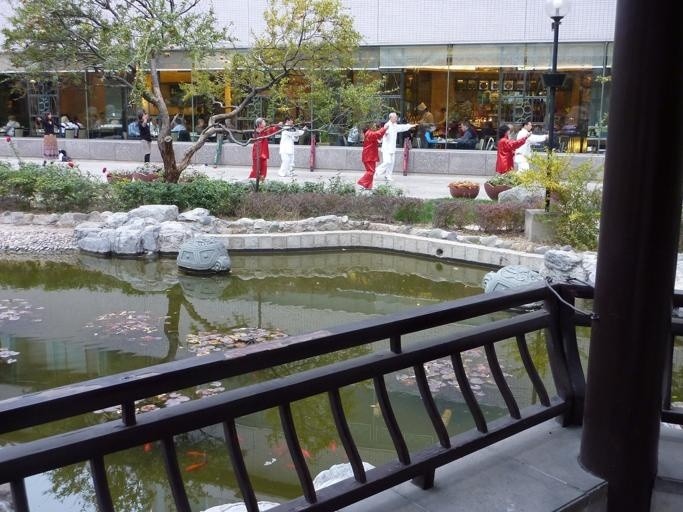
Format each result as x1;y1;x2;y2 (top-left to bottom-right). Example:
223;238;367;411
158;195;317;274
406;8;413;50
301;446;310;458
143;444;153;455
182;462;204;474
187;448;207;458
328;438;338;452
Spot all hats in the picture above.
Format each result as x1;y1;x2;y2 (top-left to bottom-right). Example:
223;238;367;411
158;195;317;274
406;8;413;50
416;102;427;112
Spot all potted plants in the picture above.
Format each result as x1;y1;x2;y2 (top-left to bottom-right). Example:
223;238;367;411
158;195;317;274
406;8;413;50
484;167;516;200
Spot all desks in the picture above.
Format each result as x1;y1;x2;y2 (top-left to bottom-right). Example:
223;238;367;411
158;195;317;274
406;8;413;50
433;138;458;149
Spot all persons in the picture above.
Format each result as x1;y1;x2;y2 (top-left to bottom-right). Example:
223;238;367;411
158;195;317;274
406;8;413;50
247;118;283;179
136;108;152;140
170;118;186;138
417;107;434;123
375;113;418;181
513;118;548;172
356;123;388;190
437;106;449;125
194;117;205;135
42;110;64;138
494;124;531;173
278;115;307;177
453;120;475;149
0;113;20;136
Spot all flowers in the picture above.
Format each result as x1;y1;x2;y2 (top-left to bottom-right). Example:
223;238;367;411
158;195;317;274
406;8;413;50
450;181;479;190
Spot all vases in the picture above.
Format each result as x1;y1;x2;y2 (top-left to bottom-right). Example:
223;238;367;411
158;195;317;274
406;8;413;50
450;188;479;198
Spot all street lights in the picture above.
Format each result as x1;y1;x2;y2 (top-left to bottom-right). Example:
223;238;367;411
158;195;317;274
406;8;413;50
546;0;567;211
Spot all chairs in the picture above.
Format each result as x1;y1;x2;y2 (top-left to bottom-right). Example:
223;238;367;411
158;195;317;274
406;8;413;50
0;125;197;141
416;138;422;148
479;138;485;150
342;134;348;146
